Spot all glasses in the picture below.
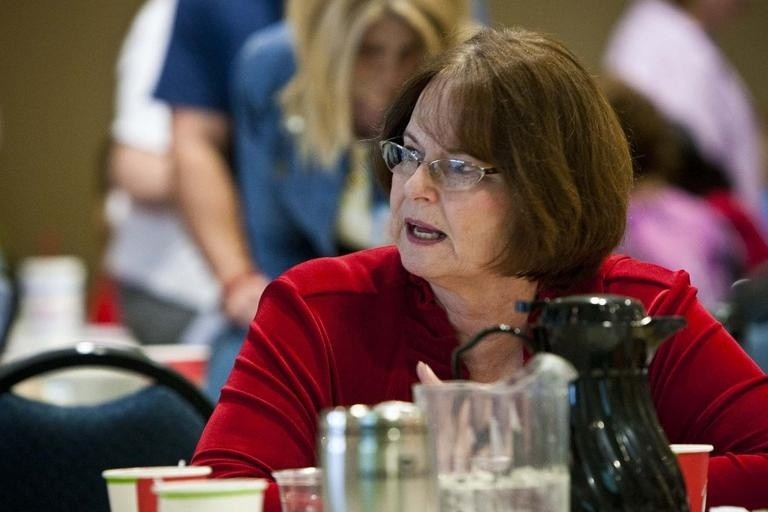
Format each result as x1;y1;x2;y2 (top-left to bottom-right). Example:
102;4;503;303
380;133;501;192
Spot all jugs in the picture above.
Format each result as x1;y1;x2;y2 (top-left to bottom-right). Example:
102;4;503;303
412;350;579;512
450;295;690;512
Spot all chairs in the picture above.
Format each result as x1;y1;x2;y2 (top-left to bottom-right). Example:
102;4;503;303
0;346;214;512
714;274;768;374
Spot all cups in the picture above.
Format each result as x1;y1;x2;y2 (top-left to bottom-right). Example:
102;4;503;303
271;467;320;512
321;400;428;512
102;466;214;511
151;474;268;511
138;343;208;392
669;443;715;512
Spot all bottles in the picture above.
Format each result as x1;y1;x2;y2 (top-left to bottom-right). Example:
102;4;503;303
1;253;91;371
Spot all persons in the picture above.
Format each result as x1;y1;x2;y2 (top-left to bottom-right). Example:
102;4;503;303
194;25;768;512
110;0;474;398
592;1;768;369
